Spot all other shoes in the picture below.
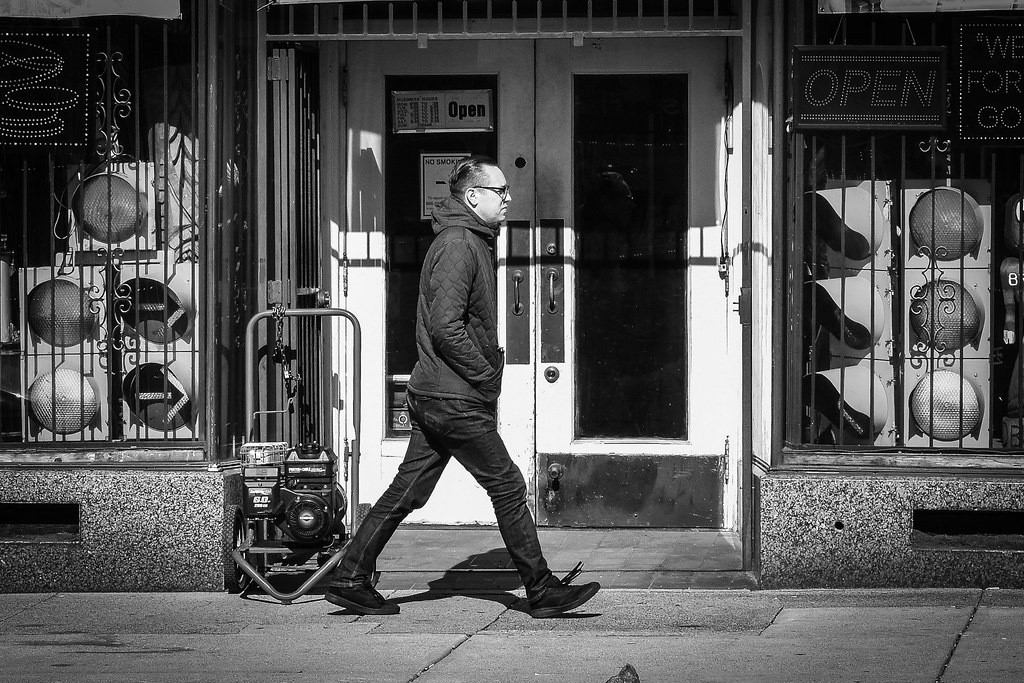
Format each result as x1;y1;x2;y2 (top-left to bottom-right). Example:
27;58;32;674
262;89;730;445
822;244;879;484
528;575;601;618
325;585;400;614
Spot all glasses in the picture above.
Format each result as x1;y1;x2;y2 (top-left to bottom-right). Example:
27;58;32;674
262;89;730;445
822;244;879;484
618;196;636;204
472;185;510;198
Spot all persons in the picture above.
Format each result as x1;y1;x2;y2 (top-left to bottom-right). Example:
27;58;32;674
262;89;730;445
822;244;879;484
324;158;601;618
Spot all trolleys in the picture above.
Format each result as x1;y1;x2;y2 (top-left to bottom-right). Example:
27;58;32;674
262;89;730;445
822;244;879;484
220;307;374;606
